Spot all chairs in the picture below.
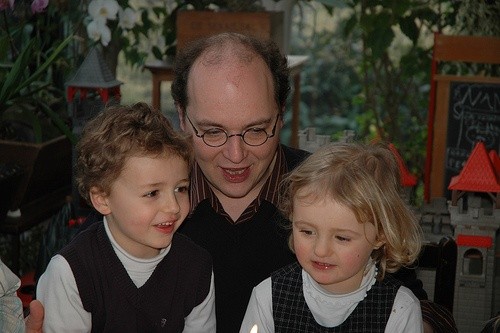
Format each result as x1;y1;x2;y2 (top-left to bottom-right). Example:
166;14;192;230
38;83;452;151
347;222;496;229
417;236;458;317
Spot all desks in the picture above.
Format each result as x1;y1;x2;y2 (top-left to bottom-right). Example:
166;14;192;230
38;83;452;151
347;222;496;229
143;55;310;148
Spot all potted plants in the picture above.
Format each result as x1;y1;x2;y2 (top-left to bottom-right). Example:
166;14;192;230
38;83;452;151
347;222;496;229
0;27;79;233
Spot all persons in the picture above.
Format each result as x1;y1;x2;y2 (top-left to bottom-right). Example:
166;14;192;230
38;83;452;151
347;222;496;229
36;101;218;333
24;31;457;333
238;142;426;333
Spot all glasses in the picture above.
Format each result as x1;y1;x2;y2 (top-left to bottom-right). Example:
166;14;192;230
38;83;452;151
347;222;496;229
183;108;279;147
